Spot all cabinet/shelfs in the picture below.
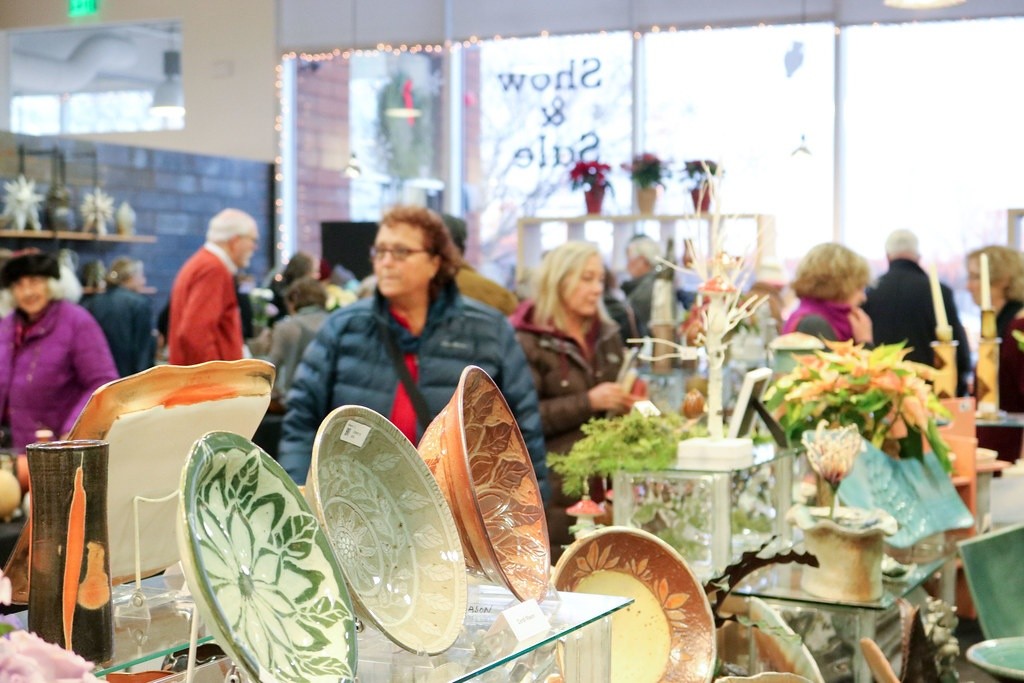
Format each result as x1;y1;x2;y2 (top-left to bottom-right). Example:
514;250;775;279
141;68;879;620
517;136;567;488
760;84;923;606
0;229;158;296
516;216;776;282
560;397;980;683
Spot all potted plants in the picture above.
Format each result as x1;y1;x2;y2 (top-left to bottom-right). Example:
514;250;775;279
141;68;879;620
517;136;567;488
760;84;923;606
752;330;955;473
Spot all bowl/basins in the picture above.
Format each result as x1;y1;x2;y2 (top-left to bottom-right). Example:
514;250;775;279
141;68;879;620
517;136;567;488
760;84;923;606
552;525;718;683
305;405;469;656
414;363;551;604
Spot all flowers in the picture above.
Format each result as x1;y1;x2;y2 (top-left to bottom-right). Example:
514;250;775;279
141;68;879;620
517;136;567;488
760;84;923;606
801;419;861;523
678;159;719;186
569;161;615;199
621;153;673;191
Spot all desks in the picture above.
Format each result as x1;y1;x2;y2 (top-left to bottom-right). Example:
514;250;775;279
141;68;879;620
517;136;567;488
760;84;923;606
976;414;1023;477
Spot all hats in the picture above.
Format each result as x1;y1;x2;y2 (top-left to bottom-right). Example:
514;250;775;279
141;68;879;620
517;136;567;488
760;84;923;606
0;248;60;288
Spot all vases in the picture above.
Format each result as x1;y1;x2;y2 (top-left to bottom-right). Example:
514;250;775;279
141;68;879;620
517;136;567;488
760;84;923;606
780;504;898;604
584;192;604;214
692;189;711;212
638;188;656;214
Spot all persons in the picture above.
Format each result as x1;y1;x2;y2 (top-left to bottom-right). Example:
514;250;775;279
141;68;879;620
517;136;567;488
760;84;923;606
966;245;1024;458
438;214;519;317
158;208;358;401
601;234;660;341
863;229;971;395
783;242;874;350
278;206;552;512
513;240;639;565
0;248;159;450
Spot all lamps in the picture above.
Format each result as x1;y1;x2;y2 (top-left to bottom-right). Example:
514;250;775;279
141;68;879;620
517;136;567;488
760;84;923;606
152;26;182;111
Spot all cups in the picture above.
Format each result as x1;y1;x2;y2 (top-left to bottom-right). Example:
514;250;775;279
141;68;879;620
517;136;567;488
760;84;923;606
26;441;112;663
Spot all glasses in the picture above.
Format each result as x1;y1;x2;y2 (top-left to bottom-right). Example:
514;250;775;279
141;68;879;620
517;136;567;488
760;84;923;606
366;242;429;264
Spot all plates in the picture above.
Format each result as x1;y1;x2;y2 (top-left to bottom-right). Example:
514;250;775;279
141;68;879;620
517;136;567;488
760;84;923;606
175;429;359;683
2;359;278;604
